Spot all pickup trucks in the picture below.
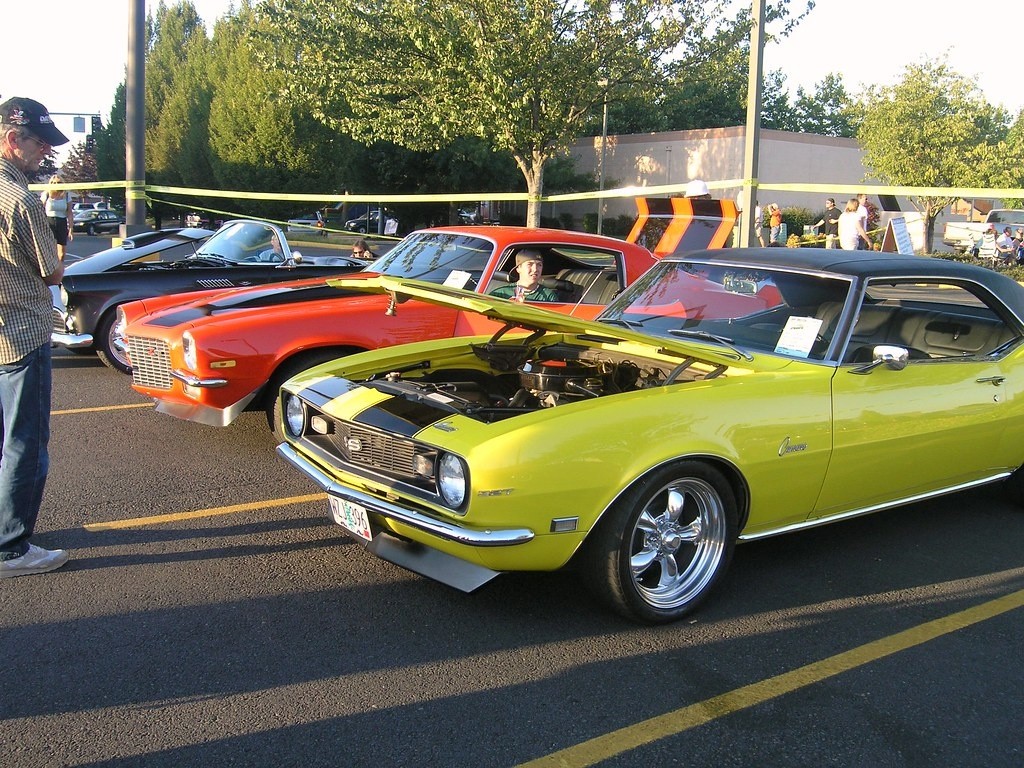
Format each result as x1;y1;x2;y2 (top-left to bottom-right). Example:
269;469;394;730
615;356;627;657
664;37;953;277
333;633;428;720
943;209;1023;248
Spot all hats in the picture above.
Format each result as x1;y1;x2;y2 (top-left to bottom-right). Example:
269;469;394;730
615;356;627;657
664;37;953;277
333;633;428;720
0;97;69;146
828;198;835;206
684;179;709;198
510;247;544;278
772;203;778;210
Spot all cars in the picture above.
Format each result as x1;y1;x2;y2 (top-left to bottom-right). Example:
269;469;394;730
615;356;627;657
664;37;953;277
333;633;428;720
49;220;484;379
73;202;127;237
458;208;476;224
273;247;1024;625
344;211;387;233
111;226;780;429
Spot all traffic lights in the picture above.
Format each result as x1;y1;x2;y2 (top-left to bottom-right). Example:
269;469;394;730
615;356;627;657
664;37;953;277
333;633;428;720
92;117;102;131
86;134;94;153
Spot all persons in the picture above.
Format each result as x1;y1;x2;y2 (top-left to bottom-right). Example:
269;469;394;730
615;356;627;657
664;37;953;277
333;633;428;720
351;240;378;258
838;199;872;251
971;227;1024;265
767;203;781;243
0;97;68;577
40;175;74;261
755;200;765;247
813;198;842;249
488;248;559;302
856;194;869;250
258;235;302;262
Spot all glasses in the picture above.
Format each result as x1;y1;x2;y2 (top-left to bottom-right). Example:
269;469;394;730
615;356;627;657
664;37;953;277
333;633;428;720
5;128;51;154
354;250;363;254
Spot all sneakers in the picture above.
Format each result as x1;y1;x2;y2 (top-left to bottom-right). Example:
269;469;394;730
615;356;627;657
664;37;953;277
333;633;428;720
0;542;68;578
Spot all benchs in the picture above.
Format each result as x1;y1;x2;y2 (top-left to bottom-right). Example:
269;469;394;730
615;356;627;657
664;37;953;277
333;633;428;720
815;298;1017;363
554;269;619;305
